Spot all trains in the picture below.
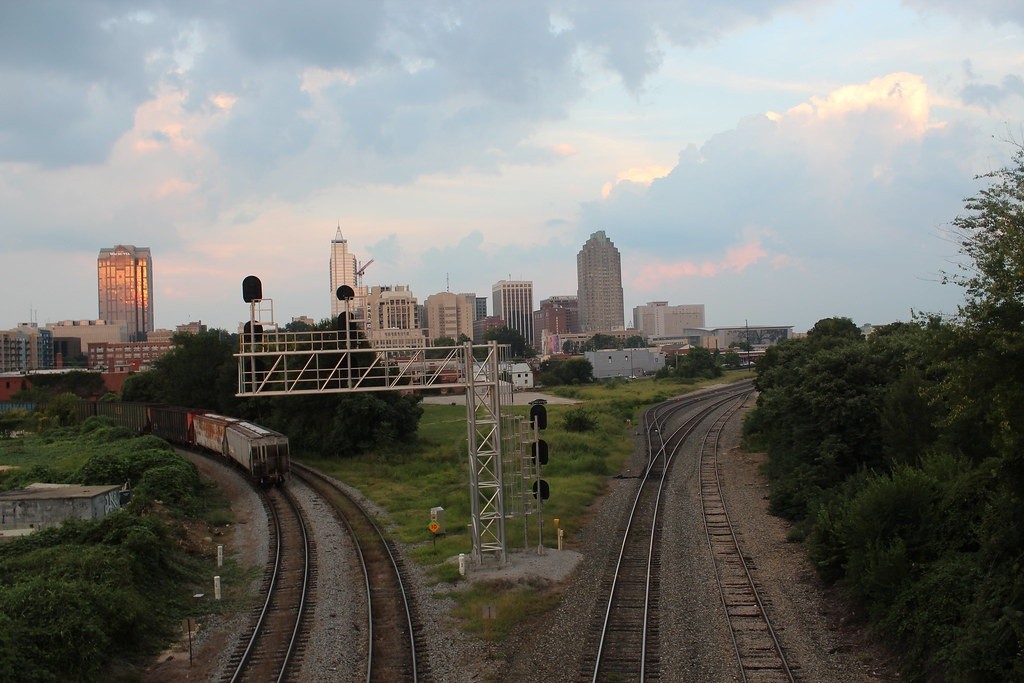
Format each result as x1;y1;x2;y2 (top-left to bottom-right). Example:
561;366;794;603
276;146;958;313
0;398;291;488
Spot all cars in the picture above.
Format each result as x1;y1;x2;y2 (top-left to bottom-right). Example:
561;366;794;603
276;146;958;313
720;361;756;370
